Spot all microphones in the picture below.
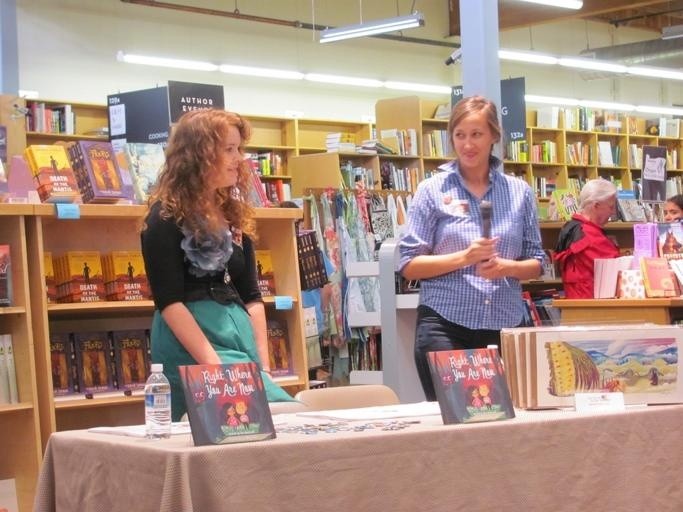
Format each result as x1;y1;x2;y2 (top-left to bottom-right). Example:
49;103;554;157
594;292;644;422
479;200;492;262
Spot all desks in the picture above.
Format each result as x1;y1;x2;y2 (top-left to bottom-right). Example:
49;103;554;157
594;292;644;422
28;388;682;512
549;289;683;329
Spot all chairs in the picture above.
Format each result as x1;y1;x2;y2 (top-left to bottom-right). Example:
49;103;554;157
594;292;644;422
291;385;404;414
179;398;312;425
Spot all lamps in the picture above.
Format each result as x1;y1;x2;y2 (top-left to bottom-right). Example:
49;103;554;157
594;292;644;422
317;0;683;81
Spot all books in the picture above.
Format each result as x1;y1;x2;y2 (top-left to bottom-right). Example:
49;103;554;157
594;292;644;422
43;250;279;305
50;321;293;395
1;245;19;512
521;249;560;327
594;223;683;297
427;349;515;425
327;101;450;193
24;142;291;204
27;101;75;135
510;106;683;221
177;362;277;445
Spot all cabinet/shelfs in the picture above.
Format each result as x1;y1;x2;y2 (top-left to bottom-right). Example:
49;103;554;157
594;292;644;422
1;90;110;162
224;109;298;203
33;202;311;461
0;204;48;512
298;88;682;295
337;232;430;404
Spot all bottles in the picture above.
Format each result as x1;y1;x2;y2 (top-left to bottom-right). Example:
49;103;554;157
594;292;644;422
143;363;171;442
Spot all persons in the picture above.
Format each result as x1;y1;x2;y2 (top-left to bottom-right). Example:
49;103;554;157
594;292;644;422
395;96;546;404
141;106;295;424
553;179;622;299
